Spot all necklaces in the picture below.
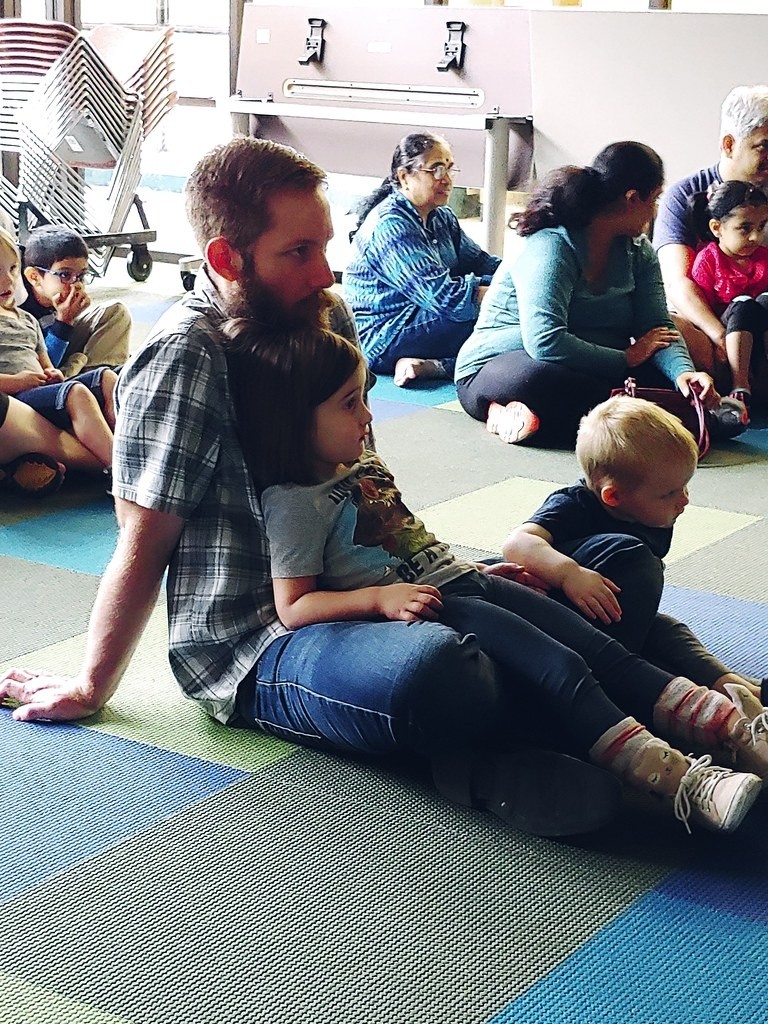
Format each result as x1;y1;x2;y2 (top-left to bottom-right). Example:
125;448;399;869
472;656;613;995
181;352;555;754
687;180;768;423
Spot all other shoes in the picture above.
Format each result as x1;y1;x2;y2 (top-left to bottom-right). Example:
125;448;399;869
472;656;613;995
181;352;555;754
0;451;66;499
486;401;540;444
703;395;748;439
102;464;112;496
718;388;752;425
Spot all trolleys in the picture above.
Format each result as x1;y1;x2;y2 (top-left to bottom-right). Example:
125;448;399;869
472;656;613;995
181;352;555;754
14;194;156;282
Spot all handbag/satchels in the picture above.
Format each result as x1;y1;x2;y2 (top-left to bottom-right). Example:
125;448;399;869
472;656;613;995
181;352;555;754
610;377;709;461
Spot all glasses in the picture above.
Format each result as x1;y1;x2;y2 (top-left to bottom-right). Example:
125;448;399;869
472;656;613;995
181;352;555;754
34;264;97;285
406;163;461;180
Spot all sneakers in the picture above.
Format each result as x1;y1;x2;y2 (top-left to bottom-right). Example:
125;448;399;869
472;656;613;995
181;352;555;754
726;706;768;772
668;752;764;834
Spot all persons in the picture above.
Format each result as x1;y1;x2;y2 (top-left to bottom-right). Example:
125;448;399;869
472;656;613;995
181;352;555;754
227;321;768;833
0;134;768;875
455;142;748;443
503;395;768;708
342;133;503;386
652;85;768;364
0;205;132;495
